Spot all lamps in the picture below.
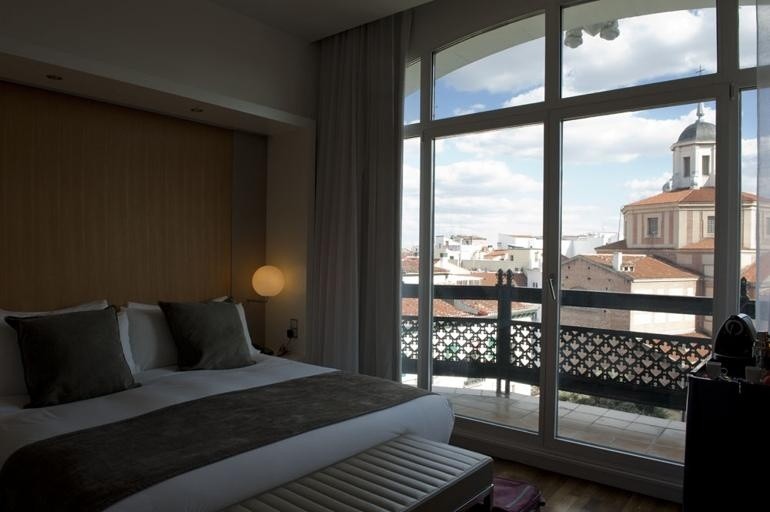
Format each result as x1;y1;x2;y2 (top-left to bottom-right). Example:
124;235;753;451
246;266;284;303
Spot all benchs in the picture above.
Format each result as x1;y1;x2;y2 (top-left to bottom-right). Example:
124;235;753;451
216;434;495;511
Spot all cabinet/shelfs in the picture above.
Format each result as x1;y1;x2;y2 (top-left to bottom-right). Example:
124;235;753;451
683;353;770;512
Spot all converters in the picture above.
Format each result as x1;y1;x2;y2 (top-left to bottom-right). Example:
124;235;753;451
286;328;294;338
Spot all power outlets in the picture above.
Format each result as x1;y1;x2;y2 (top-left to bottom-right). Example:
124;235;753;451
290;318;298;338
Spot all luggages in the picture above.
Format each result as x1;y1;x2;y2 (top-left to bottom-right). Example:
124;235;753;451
477;477;545;512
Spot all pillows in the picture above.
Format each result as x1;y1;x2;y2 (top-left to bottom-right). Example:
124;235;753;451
5;301;259;410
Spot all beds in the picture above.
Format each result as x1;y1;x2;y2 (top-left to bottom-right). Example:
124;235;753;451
0;299;455;512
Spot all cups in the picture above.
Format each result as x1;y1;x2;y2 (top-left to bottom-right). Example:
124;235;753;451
706;361;729;378
744;366;762;384
